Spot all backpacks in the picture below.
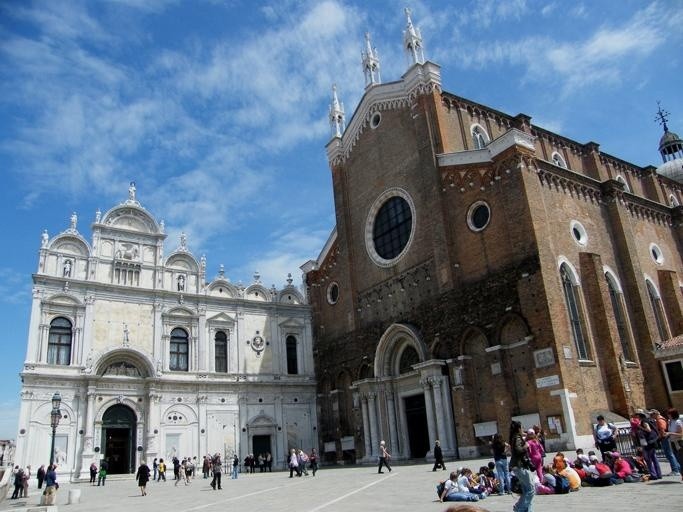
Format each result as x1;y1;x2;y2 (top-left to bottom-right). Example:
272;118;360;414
591;463;612;478
437;480;447;501
548;474;569;494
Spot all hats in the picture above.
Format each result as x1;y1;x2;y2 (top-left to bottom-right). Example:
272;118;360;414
576;450;620;461
527;428;534;435
635;409;646;417
648;409;661;415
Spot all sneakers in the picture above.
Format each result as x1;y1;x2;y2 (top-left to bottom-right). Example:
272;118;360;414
666;470;680;476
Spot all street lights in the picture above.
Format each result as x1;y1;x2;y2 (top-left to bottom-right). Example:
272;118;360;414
40;390;63;506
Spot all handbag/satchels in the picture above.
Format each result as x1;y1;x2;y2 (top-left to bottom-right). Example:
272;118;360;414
528;460;535;472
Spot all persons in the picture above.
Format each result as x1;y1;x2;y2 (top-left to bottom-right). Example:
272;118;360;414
90;463;97;482
288;448;319;477
153;453;223;490
40;491;47;505
37;465;45;488
11;465;31;499
44;465;57;506
232;454;238;479
378;440;392;473
136;460;151;496
244;452;271;472
98;459;108;486
433;408;683;512
